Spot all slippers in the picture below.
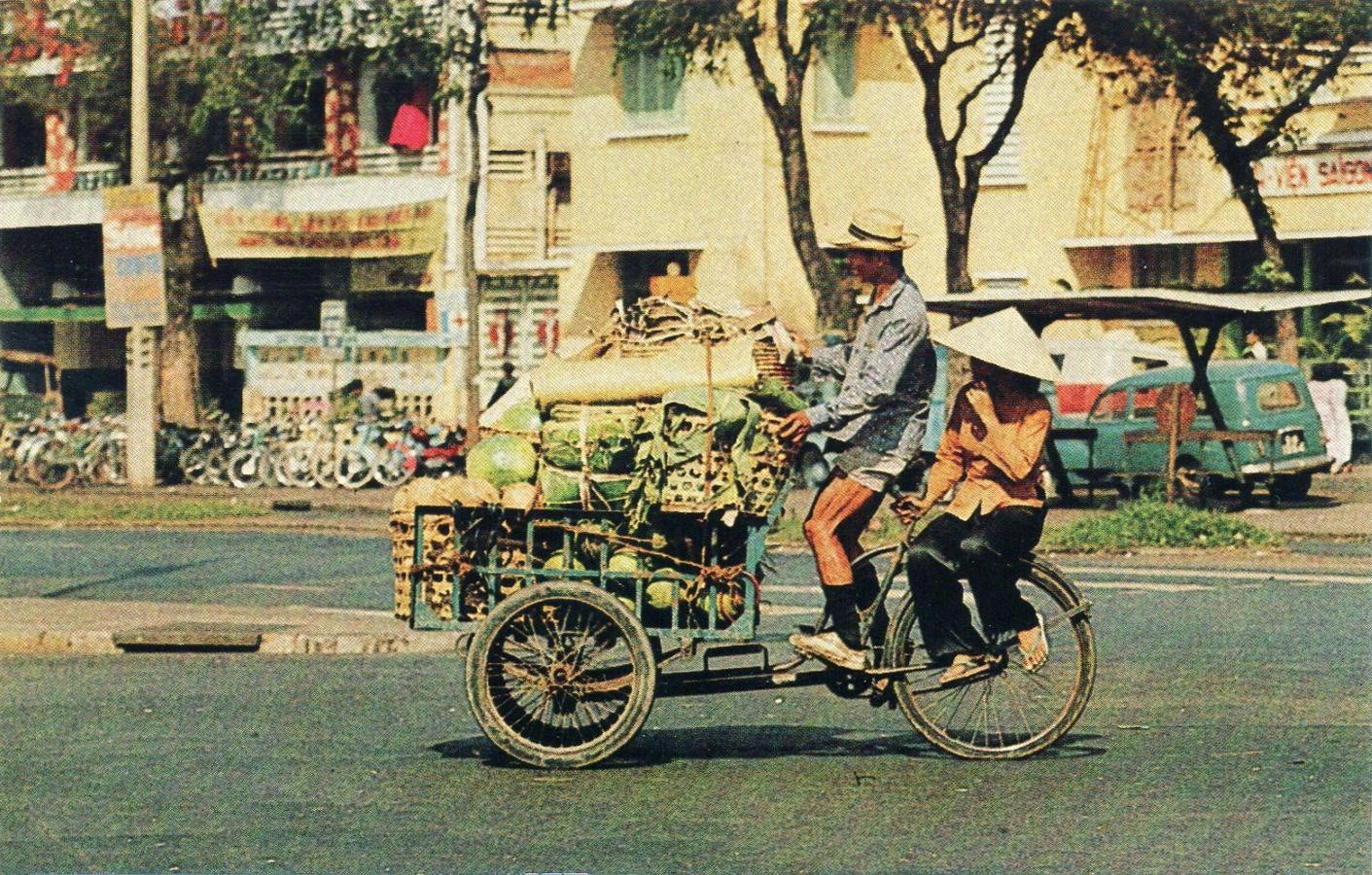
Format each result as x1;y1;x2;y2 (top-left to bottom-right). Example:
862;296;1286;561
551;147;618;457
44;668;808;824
938;654;999;684
1017;613;1049;671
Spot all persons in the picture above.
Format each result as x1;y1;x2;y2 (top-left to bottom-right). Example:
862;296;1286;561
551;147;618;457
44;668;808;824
1307;361;1354;475
890;305;1057;686
1238;328;1268;362
775;206;935;670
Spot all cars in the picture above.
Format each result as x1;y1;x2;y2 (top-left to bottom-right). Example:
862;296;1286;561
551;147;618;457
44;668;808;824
1039;360;1336;508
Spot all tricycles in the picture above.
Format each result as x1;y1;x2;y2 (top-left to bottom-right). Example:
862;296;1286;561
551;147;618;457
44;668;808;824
408;417;1099;770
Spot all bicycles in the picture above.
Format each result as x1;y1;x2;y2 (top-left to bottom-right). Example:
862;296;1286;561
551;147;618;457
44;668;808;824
0;400;464;492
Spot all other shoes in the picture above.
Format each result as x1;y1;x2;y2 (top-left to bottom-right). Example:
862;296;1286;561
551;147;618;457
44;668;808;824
789;631;865;670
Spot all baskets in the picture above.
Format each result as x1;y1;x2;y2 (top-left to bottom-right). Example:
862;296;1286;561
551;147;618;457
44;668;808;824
662;411;805;523
390;514;526;625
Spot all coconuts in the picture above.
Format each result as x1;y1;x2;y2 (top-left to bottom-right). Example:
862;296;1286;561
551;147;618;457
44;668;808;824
394;407;743;623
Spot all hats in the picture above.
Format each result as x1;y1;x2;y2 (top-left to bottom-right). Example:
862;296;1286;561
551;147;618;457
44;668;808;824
925;304;1062;382
826;209;919;252
